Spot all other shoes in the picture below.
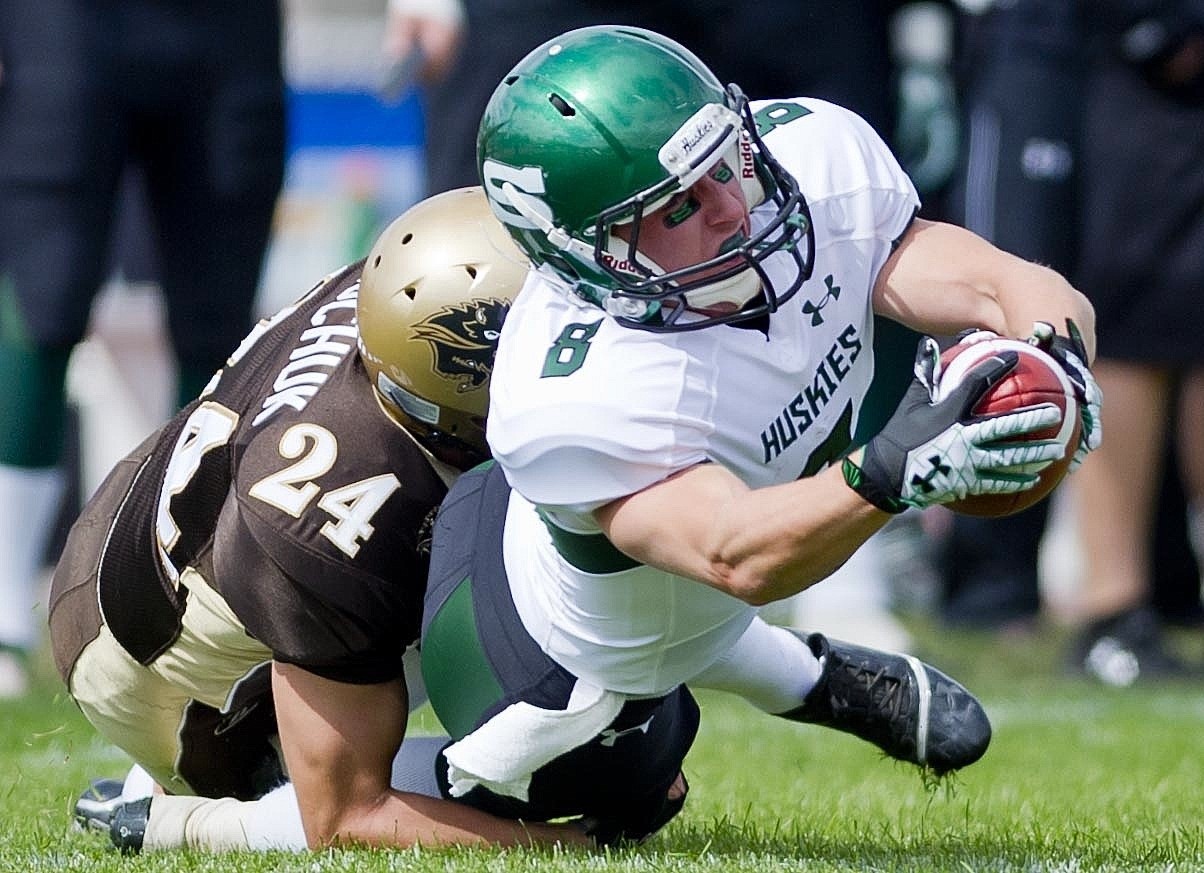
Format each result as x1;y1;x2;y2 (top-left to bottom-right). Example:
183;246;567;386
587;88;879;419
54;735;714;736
1068;612;1175;692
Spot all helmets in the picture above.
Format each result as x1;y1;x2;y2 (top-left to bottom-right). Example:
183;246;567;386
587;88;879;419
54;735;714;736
476;28;776;327
354;186;535;437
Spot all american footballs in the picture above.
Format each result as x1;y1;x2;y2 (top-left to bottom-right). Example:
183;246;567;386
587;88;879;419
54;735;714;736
938;337;1081;519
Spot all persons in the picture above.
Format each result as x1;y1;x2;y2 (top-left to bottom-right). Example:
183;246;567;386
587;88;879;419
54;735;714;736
0;0;1203;684
50;187;688;854
76;25;1104;855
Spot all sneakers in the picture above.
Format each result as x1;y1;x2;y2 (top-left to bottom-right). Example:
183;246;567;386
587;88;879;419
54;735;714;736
74;780;154;857
772;627;992;774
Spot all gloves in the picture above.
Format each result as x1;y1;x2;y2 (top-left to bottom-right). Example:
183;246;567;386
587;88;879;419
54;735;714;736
841;335;1065;518
1025;320;1104;478
